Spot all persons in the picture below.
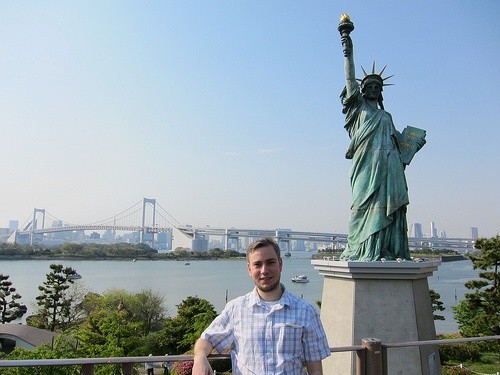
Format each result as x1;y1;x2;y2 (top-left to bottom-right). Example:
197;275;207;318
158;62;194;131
192;237;331;375
338;36;427;263
144;354;155;375
163;354;170;375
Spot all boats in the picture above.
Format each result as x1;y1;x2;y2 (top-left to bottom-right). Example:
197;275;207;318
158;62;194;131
291;274;310;283
63;271;82;280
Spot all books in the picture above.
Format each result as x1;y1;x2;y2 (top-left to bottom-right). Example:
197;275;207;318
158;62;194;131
399;126;426;165
